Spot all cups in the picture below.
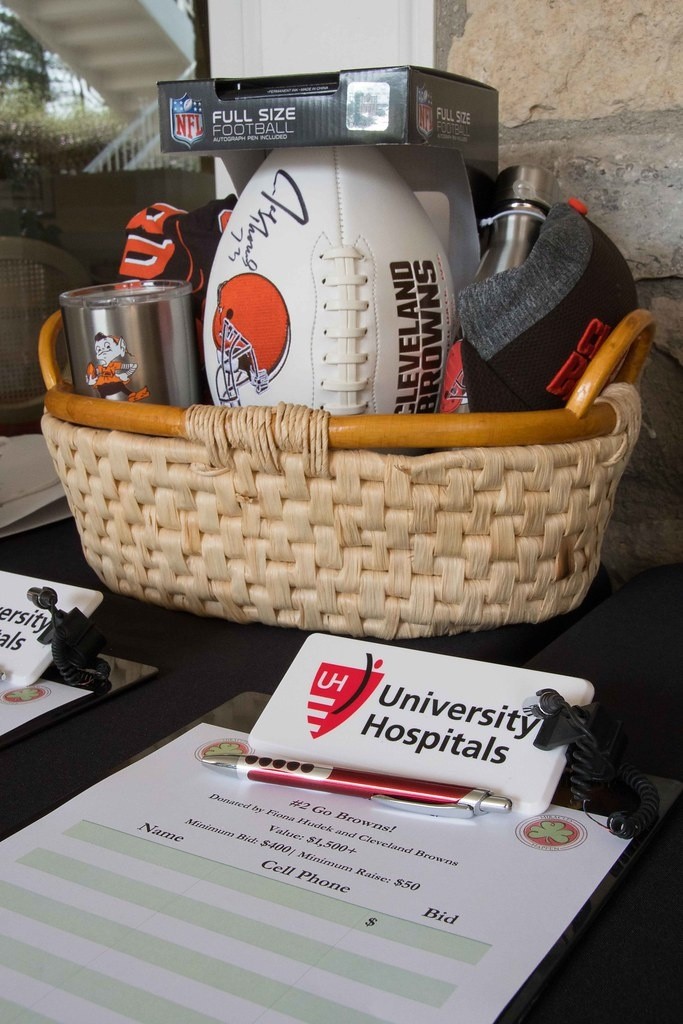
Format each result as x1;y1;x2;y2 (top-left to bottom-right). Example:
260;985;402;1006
58;281;203;413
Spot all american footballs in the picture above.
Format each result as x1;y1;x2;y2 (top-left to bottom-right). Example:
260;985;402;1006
202;143;456;457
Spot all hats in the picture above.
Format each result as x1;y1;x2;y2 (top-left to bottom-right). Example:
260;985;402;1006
455;194;641;417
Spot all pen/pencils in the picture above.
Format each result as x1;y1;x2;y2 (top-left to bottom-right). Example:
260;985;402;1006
200;754;513;820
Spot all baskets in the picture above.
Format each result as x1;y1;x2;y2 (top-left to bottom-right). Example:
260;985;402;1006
35;302;655;640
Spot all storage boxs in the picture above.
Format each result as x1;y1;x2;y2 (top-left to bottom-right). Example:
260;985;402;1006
32;308;661;642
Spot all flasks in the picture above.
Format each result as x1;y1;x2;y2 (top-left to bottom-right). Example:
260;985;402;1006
473;163;561;283
